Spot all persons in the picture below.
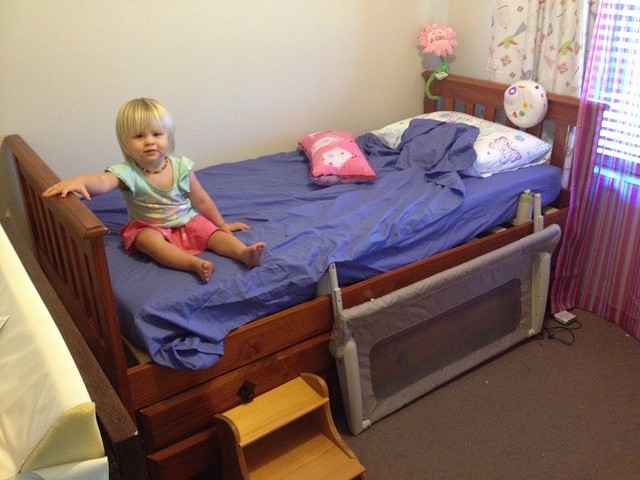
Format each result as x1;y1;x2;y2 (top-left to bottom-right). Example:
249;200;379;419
42;97;265;283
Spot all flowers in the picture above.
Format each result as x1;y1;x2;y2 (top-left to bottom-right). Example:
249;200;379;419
416;22;458;100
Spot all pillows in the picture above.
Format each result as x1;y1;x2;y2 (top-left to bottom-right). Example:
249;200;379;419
297;128;377;188
371;109;556;180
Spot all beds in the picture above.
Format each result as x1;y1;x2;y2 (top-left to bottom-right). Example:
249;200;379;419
0;217;151;479
4;69;610;480
